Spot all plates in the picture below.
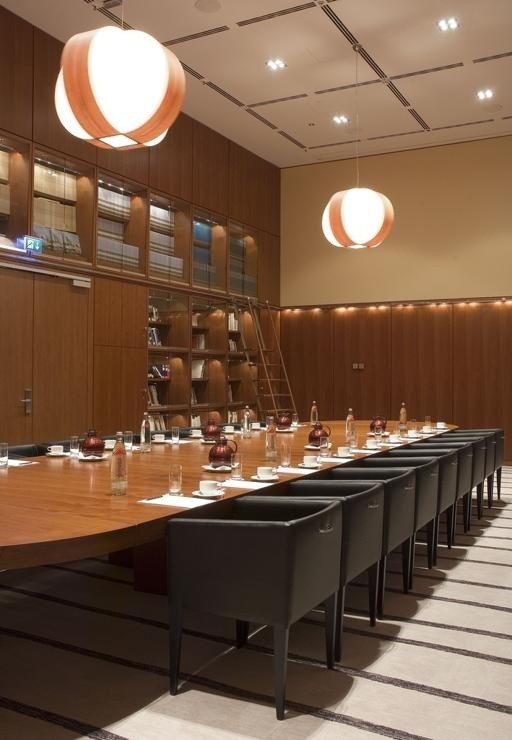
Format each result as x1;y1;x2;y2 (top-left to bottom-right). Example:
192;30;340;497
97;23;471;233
334;454;355;458
298;463;322;469
48;453;69;456
385;441;402;444
362;447;380;450
192;490;225;497
251;476;278;481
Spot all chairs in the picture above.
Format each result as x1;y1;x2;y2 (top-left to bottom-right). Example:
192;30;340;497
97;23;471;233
304;468;417;618
0;421;274;462
162;495;342;721
243;479;386;658
374;426;502;563
335;457;440;589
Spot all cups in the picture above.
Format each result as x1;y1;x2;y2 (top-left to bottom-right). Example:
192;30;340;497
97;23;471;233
319;437;329;457
230;452;242;480
374;426;382;443
199;481;222;496
280;438;291;466
408;416;449;439
303;455;321;465
0;443;8;468
398;425;408;437
170;463;183;496
366;440;379;448
104;440;115;447
337;447;350;457
389;435;400;441
47;446;63;454
256;467;277;478
124;408;306;453
348;427;359;447
70;436;80;454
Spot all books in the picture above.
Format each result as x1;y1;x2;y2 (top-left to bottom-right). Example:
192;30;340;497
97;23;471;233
189;306;206;430
145;300;165;432
226;311;241;424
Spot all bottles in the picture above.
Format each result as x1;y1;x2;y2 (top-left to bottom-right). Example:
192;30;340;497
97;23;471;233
310;400;319;424
264;416;276;456
242;405;251;438
111;432;129;496
345;408;355;443
399;401;407;424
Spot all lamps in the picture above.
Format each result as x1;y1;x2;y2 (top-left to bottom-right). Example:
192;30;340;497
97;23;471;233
321;43;395;249
50;1;190;152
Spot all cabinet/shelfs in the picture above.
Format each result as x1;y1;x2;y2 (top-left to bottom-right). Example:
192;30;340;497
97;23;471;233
1;132;283;446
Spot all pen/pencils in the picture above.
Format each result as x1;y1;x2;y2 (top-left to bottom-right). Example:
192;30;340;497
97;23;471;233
147;496;163;501
20;462;33;465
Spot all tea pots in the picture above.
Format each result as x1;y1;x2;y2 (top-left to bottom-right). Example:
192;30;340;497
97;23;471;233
207;437;238;467
78;430;105;457
310;423;331;446
369;415;387;434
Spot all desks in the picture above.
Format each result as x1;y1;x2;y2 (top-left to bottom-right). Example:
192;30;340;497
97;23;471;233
0;415;458;575
